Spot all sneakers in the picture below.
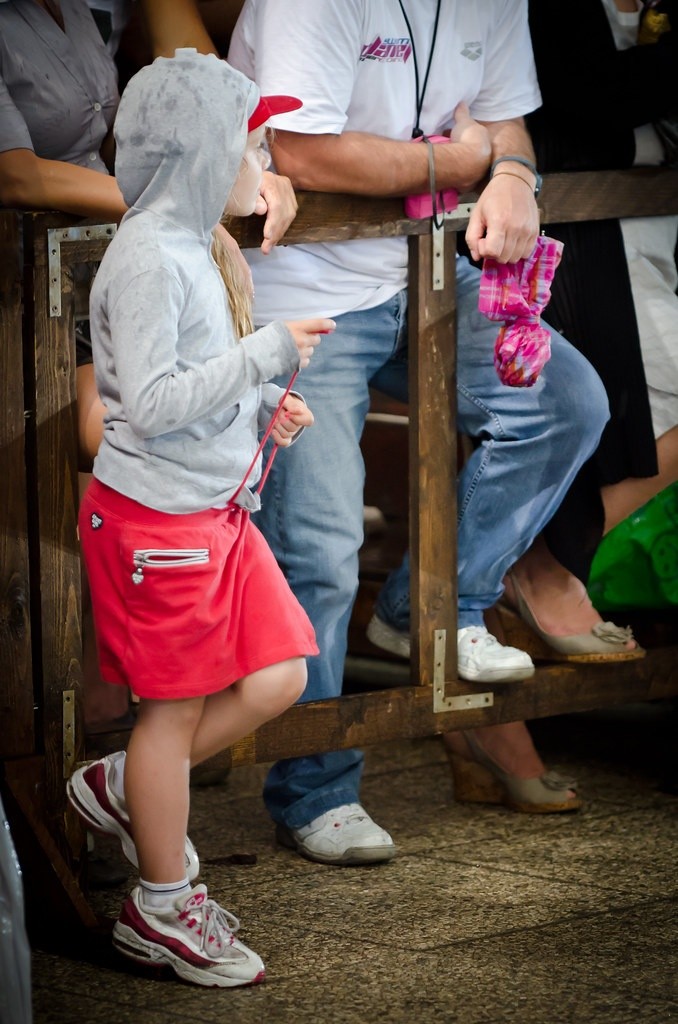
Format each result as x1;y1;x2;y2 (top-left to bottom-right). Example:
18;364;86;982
367;613;536;682
110;886;264;989
66;751;200;883
276;801;396;865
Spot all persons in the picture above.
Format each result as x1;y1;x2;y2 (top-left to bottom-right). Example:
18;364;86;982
443;0;678;813
64;47;320;987
226;0;611;861
0;0;299;723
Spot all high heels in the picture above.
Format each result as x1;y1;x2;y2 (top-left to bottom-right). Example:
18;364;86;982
446;729;582;813
493;564;645;664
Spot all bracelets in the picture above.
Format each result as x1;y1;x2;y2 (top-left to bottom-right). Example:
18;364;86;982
493;172;534;192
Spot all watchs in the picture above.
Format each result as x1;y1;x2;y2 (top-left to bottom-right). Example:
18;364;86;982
490;156;543;198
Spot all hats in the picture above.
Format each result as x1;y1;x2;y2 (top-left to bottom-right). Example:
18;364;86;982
248;96;303;131
